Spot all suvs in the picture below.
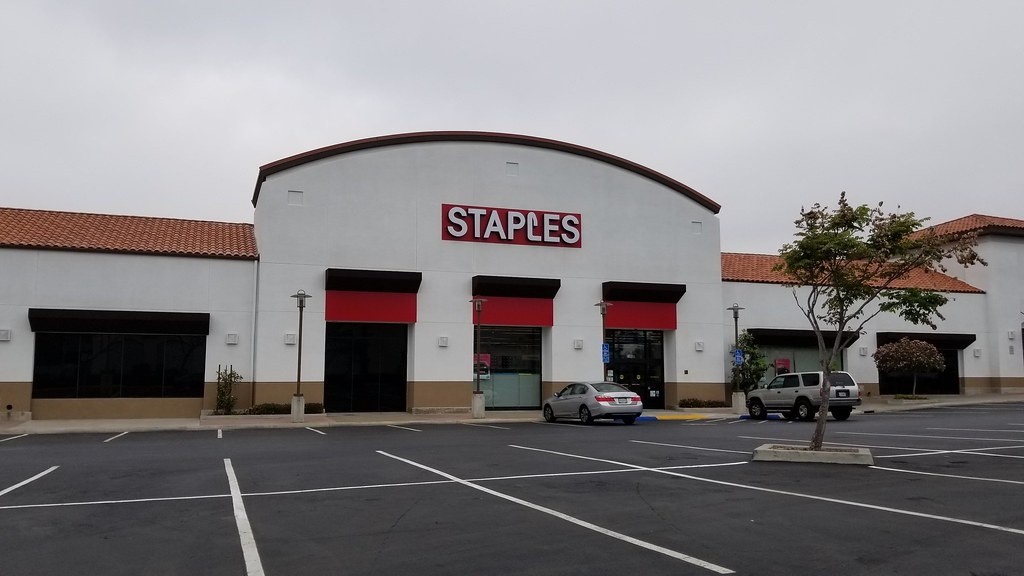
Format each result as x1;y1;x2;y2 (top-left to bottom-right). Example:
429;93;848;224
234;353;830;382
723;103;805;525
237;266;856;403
746;370;862;421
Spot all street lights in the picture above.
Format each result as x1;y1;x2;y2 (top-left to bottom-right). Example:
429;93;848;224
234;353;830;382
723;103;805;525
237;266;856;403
468;294;487;418
289;290;312;422
726;303;746;415
594;300;613;381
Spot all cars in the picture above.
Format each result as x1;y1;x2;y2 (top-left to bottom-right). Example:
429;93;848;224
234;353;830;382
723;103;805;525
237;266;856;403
542;381;642;423
